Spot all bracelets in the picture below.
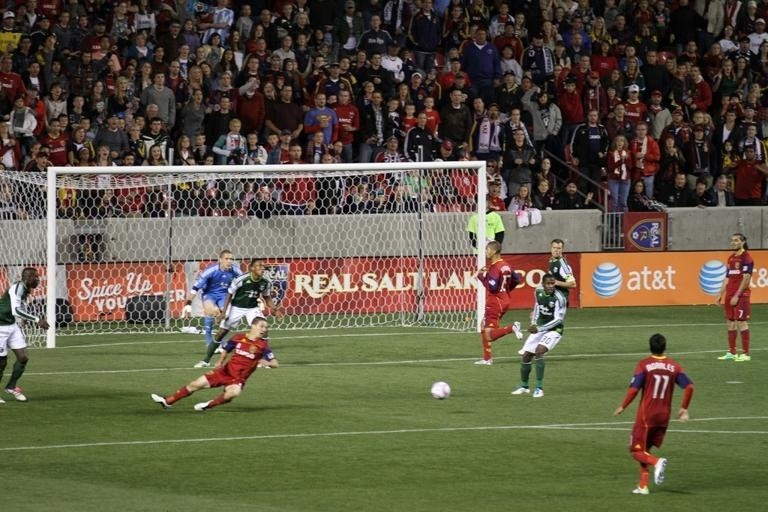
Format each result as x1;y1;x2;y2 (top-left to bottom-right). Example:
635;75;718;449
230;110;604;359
735;291;741;296
186;299;192;304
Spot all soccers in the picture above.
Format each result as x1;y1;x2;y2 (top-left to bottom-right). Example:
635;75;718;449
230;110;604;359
431;381;450;399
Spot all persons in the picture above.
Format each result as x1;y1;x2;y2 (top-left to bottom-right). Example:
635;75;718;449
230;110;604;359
182;250;245;353
150;316;278;411
474;241;523;366
0;268;50;404
0;2;768;218
510;273;566;398
547;239;577;305
467;197;504;257
195;259;270;368
615;334;694;494
718;233;753;362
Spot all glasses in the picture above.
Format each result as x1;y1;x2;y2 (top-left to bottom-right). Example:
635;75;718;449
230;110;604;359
486;163;495;169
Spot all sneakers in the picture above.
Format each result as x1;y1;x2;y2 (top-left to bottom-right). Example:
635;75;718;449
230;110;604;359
509;386;530;397
212;343;226;354
193;360;210;369
472;358;494;366
732;353;751;362
510;320;523;341
3;387;28;403
0;395;7;404
531;387;545;399
715;351;737;361
192;400;215;413
630;484;649;496
652;457;667;487
149;392;172;411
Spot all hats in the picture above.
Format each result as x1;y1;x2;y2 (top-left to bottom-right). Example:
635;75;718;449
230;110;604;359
501;69;515;77
411;71;421;78
754;17;764;24
627;84;639;93
34;150;49;158
101;112;119;123
343;0;356;9
440;140;453;150
1;10;15;20
